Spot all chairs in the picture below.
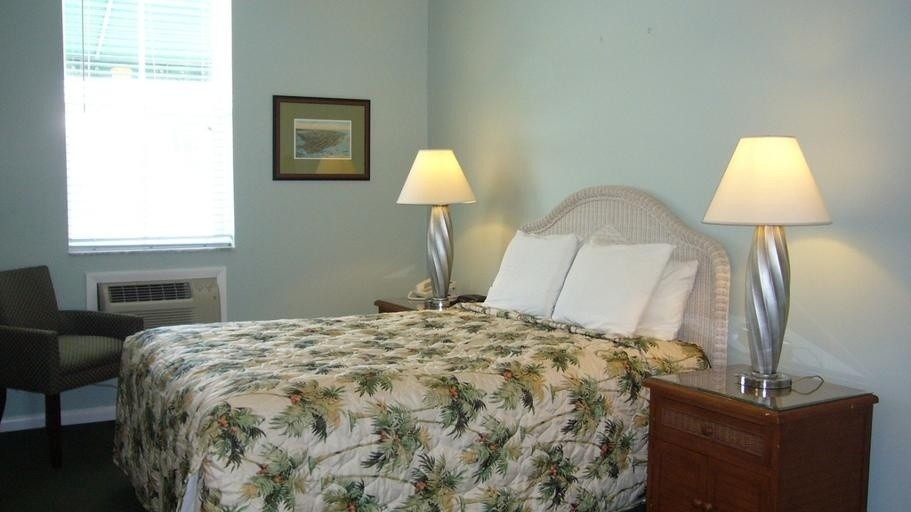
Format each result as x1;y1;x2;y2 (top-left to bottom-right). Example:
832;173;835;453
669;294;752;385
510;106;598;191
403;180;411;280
1;266;146;463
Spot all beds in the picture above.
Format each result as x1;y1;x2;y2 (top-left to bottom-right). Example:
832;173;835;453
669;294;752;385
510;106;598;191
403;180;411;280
113;184;732;511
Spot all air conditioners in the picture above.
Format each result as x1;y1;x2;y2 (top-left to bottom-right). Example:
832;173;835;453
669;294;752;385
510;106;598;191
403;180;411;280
97;276;223;374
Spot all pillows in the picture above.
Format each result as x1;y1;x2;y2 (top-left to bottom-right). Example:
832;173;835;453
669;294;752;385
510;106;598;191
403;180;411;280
552;231;679;338
483;228;586;321
632;259;701;342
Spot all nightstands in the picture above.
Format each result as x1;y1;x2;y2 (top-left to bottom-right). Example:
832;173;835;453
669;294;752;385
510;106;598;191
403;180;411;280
374;296;431;314
640;364;880;512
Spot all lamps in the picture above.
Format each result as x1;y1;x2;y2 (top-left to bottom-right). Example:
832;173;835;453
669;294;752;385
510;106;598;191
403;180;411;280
700;136;834;389
397;149;479;309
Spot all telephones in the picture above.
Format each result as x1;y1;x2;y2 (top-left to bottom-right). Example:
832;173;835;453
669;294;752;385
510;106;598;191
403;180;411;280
414;277;456;298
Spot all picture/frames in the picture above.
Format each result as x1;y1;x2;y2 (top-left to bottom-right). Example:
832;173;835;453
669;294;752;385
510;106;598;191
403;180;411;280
272;94;373;182
